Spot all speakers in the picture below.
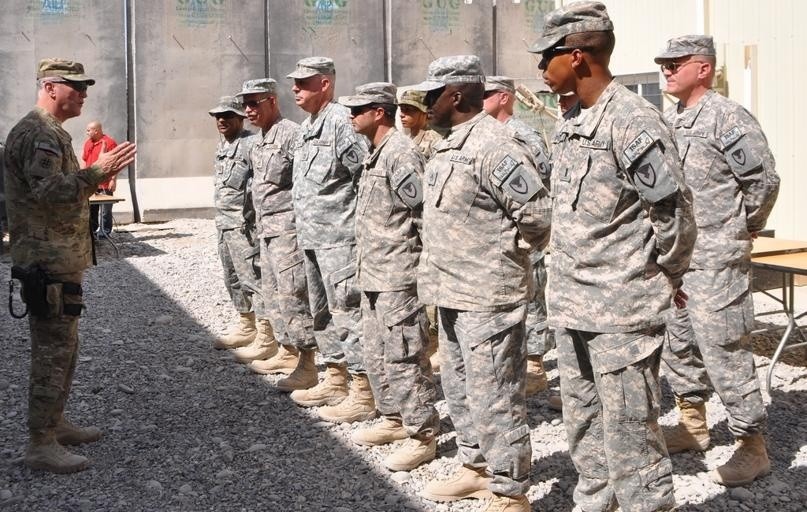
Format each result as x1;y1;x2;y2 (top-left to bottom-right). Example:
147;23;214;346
664;401;710;452
25;428;89;473
713;433;771;486
55;413;101;444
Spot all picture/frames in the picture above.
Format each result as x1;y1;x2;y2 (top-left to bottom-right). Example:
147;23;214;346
750;237;806;393
87;193;127;264
746;232;806;259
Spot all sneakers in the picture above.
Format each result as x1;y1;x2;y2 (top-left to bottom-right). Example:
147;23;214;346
422;465;494;501
485;494;531;511
384;437;436;471
351;418;410;446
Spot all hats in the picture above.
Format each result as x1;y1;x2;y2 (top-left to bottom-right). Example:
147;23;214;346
209;96;248;118
235;78;279;96
37;58;95;85
528;0;614;53
654;35;716;64
286;55;514;107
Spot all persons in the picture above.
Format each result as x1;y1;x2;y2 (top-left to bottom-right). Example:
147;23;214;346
272;56;372;424
209;95;257;350
231;76;301;374
482;76;554;397
655;35;781;486
337;81;438;471
401;55;553;512
551;90;580;410
398;90;441;161
82;121;118;239
3;58;137;475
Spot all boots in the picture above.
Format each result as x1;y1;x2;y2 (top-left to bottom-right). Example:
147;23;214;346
318;374;375;423
664;401;710;452
713;433;771;486
292;362;350;406
277;351;318;391
55;413;101;444
216;314;258;349
426;334;440;373
233;320;279;363
25;428;89;473
525;355;545;396
250;344;299;374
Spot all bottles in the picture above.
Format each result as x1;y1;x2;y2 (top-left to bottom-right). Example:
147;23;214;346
37;58;95;85
654;35;716;64
286;55;514;107
235;78;279;96
209;96;248;118
528;0;614;53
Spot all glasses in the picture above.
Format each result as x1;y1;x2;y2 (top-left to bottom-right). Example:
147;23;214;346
69;82;87;90
351;107;371;115
241;98;270;108
544;46;593;59
661;60;703;71
214;112;235;119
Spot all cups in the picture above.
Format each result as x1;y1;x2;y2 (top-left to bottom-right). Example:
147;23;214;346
661;60;703;71
69;82;87;90
214;112;235;119
544;46;593;59
351;107;371;115
241;98;270;108
